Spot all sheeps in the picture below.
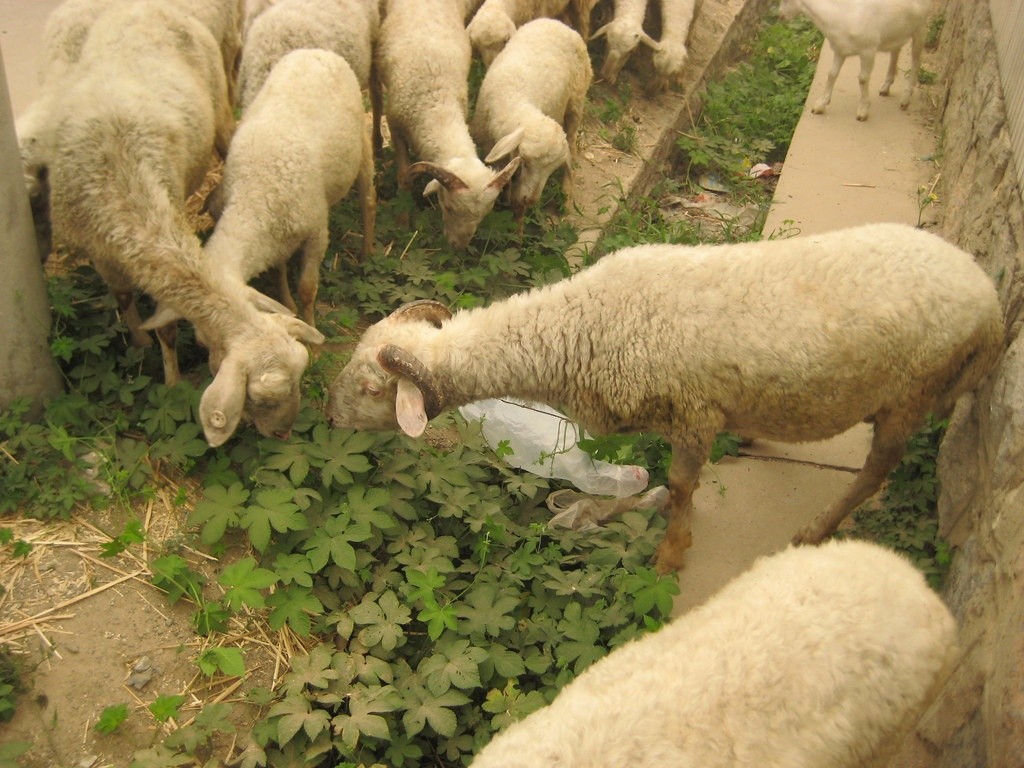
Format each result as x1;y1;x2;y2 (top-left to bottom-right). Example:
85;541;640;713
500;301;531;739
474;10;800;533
773;0;929;123
0;2;696;447
324;223;1008;579
467;537;959;768
